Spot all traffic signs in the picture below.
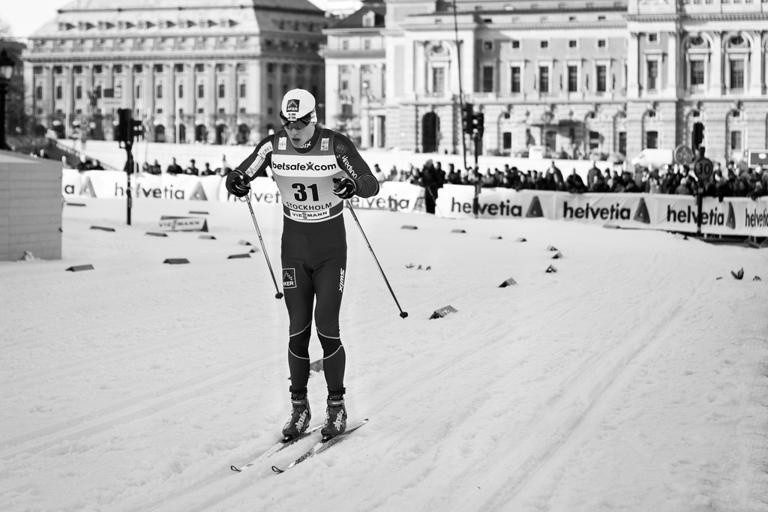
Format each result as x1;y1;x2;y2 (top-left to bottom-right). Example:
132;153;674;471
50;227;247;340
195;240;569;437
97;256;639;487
694;159;712;180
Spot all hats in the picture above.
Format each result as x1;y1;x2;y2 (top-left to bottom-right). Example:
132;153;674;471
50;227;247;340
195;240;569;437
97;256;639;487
281;89;317;130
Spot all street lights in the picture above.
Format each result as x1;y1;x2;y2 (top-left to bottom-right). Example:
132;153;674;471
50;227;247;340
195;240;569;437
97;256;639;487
496;110;595;161
612;111;700;151
723;109;740;167
0;49;16;150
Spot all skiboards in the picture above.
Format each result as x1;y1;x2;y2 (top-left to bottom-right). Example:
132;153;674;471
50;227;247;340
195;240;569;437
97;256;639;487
231;417;369;472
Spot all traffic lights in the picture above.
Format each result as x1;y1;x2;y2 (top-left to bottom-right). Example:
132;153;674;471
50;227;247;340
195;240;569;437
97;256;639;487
462;104;483;141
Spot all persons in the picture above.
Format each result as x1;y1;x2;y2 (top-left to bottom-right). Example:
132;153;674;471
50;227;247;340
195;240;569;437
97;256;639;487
29;148;106;172
123;159;233;177
225;88;379;438
369;159;767;215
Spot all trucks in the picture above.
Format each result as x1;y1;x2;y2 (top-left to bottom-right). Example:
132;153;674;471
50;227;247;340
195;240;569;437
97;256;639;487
632;148;672;171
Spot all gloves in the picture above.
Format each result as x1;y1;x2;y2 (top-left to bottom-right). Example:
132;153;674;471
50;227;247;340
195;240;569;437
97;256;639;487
226;170;250;197
331;177;356;200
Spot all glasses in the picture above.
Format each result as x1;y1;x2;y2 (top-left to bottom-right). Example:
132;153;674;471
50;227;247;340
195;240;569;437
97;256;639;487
280;112;313;130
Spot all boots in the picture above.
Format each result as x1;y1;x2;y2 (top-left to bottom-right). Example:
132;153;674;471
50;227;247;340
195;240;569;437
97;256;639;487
320;395;348;441
282;394;312;443
